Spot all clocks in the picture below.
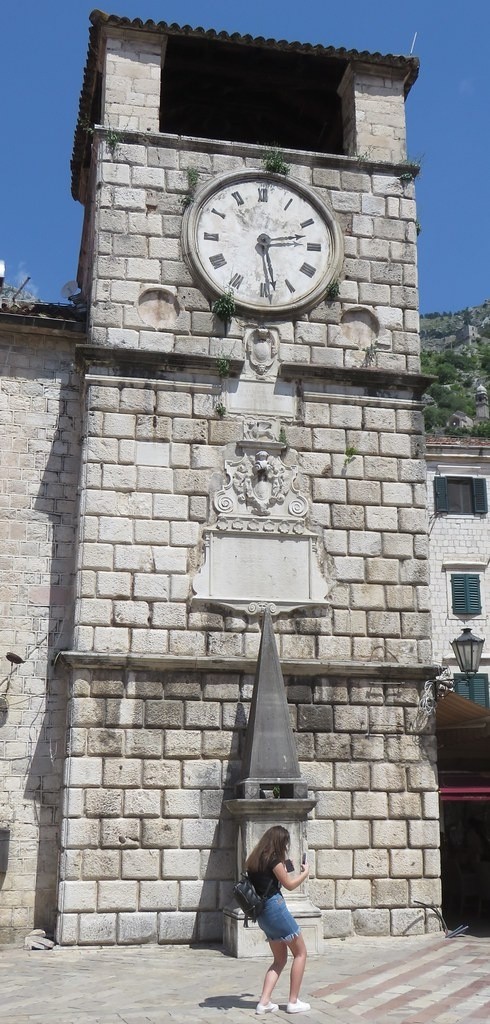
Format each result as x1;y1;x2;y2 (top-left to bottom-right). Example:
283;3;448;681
181;167;345;321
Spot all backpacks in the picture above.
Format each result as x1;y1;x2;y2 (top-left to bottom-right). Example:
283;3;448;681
234;872;274;928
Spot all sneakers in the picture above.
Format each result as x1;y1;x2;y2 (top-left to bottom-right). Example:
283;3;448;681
286;998;310;1013
256;1002;279;1014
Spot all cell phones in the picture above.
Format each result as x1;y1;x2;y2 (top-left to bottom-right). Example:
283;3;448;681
301;853;306;869
285;859;294;873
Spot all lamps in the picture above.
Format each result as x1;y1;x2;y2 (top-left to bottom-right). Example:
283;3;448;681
428;627;485;702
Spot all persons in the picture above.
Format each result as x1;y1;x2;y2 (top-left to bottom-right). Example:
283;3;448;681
244;825;311;1015
